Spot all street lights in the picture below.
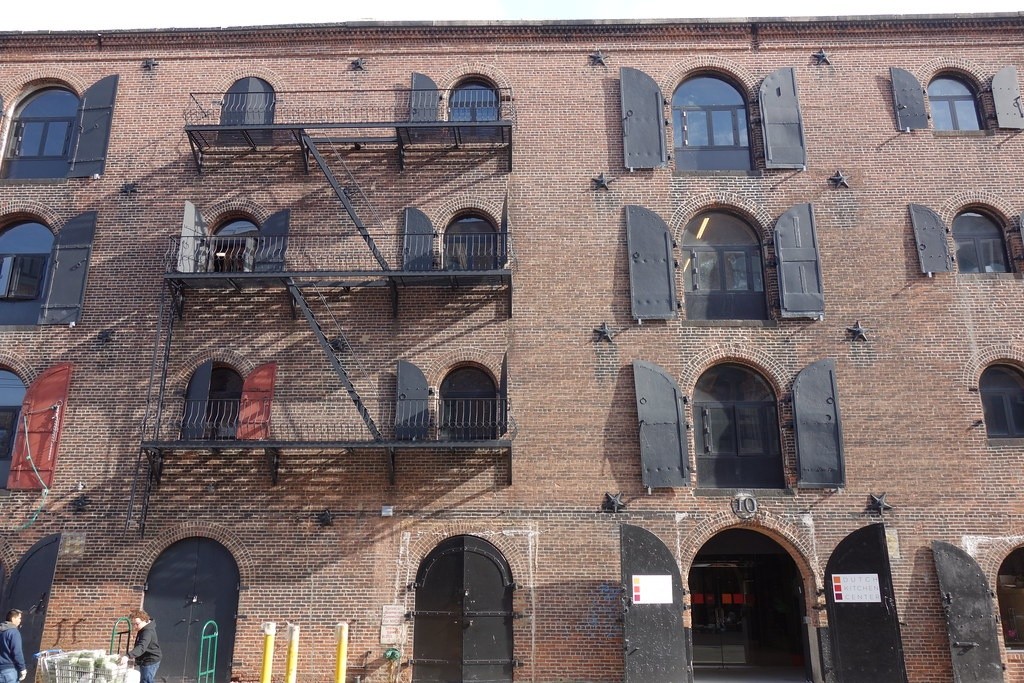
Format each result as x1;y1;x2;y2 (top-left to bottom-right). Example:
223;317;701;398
743;580;754;663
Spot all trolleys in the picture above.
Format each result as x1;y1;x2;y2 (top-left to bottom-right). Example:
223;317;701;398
33;650;142;683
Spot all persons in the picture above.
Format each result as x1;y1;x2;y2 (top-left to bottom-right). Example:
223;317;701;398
0;609;27;683
116;610;162;683
